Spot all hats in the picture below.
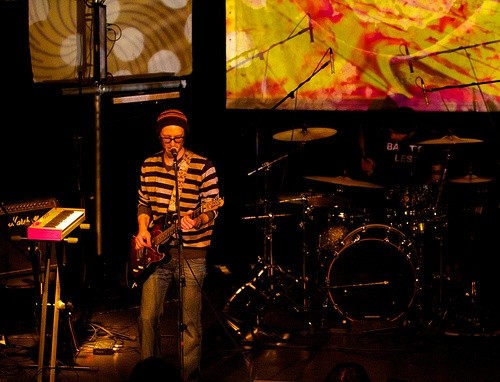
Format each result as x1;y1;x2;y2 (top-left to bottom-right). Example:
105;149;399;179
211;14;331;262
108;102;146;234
156;108;189;134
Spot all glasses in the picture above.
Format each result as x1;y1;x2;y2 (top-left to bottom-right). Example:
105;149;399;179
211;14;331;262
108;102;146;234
160;135;187;143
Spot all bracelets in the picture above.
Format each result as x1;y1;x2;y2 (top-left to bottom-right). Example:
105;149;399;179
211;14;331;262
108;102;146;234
193;216;203;230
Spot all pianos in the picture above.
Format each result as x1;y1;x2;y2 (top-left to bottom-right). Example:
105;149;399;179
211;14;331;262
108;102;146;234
27;206;87;243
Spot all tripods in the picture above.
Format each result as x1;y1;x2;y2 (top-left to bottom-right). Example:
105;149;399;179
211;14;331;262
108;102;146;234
222;60;331;354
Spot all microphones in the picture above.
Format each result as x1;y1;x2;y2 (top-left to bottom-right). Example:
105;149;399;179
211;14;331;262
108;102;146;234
421;78;429;104
170;147;178;166
404;45;414;73
309;17;314;43
330;48;335;73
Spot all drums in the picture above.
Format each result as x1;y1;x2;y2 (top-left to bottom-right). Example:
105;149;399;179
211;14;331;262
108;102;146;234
385;182;445;230
318;211;372;252
325;223;422;324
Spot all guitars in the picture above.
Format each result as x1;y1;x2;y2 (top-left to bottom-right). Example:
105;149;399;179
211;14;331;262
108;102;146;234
130;194;225;282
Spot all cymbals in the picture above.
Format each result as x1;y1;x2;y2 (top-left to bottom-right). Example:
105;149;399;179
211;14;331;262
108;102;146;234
450;175;495;184
302;175;386;190
272;126;337;142
276;193;348;208
415;136;485;144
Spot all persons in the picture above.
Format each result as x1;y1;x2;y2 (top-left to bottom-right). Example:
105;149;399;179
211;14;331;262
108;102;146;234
135;106;219;382
359;107;432;226
